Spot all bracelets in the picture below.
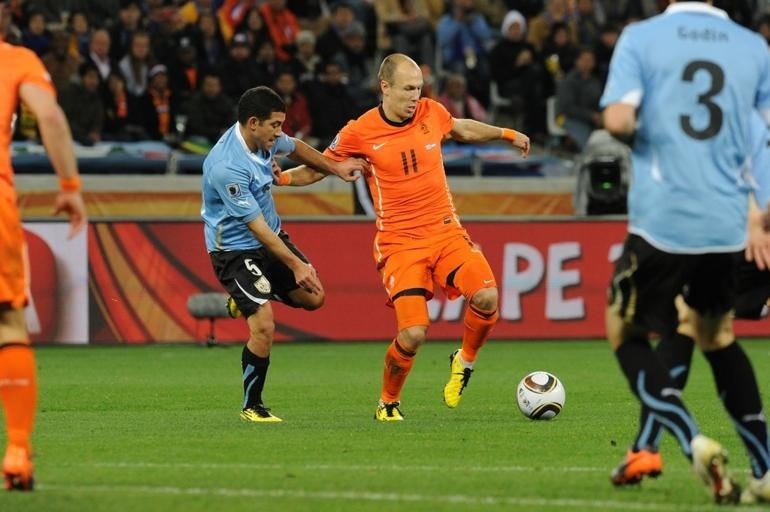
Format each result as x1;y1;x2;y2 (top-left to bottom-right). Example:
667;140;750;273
58;177;82;194
501;128;517;143
279;170;291;186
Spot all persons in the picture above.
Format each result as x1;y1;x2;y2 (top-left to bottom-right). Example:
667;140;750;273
199;84;371;425
593;0;770;503
1;22;86;493
268;54;532;423
604;230;719;488
0;1;770;218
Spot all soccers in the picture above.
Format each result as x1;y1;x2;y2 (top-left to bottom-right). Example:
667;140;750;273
517;372;564;419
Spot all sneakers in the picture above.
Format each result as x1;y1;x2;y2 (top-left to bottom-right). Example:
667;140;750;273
740;471;769;505
610;447;663;487
2;448;34;490
443;349;474;408
240;404;282;422
373;399;404;421
224;296;241;319
690;434;741;507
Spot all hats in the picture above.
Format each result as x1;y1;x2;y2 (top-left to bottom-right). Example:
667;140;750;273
502;11;527;37
